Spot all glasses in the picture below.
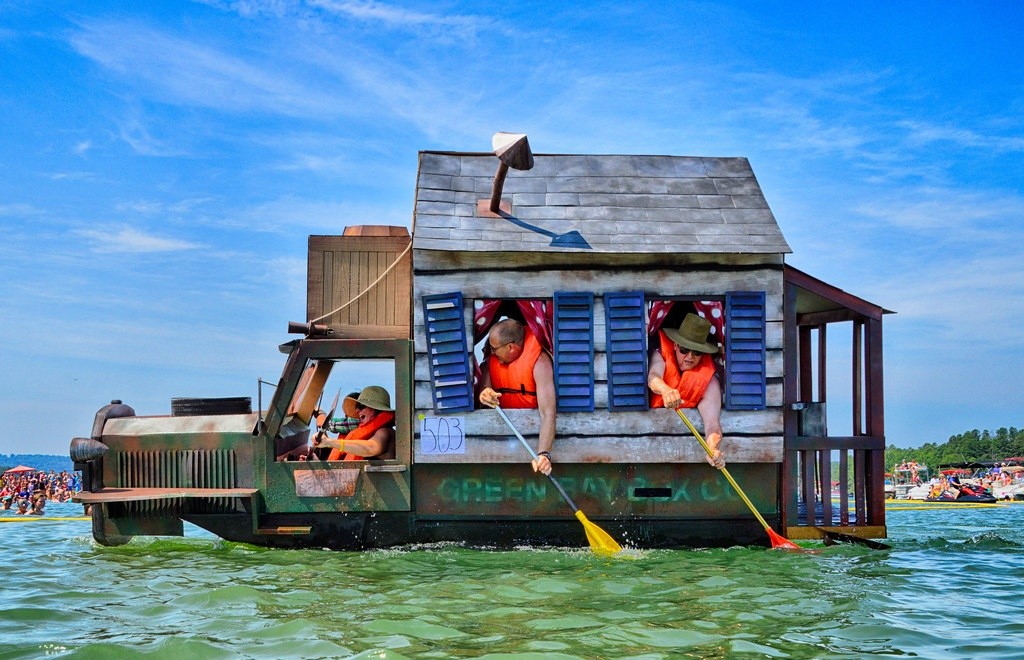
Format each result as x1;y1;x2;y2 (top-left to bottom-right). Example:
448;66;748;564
356;403;367;410
6;499;12;502
40;494;47;499
678;345;702;356
489;341;515;352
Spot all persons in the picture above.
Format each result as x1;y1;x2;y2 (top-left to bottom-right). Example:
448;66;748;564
299;386;396;461
0;470;92;517
645;313;726;470
884;459;1024;497
313;392;362;436
477;318;557;476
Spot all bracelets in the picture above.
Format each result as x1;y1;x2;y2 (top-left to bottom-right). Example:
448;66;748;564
339;439;344;452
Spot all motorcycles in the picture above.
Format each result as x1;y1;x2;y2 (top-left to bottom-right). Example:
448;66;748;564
925;482;999;502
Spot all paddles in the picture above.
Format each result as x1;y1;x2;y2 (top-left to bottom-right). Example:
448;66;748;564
496;402;622;555
674;406;801;549
307;386;341;460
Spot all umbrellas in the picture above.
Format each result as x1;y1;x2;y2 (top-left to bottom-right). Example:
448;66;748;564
5;464;36;473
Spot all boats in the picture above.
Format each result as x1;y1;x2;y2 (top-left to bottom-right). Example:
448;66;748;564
69;129;898;548
820;497;998;504
843;504;999;511
1;517;93;523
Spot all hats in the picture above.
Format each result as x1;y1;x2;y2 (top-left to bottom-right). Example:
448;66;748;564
342;386;395;419
663;313;718;353
17;499;30;504
4;496;12;500
982;462;1011;486
33;492;47;500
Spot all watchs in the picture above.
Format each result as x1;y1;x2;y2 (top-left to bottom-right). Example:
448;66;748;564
538;451;551;461
